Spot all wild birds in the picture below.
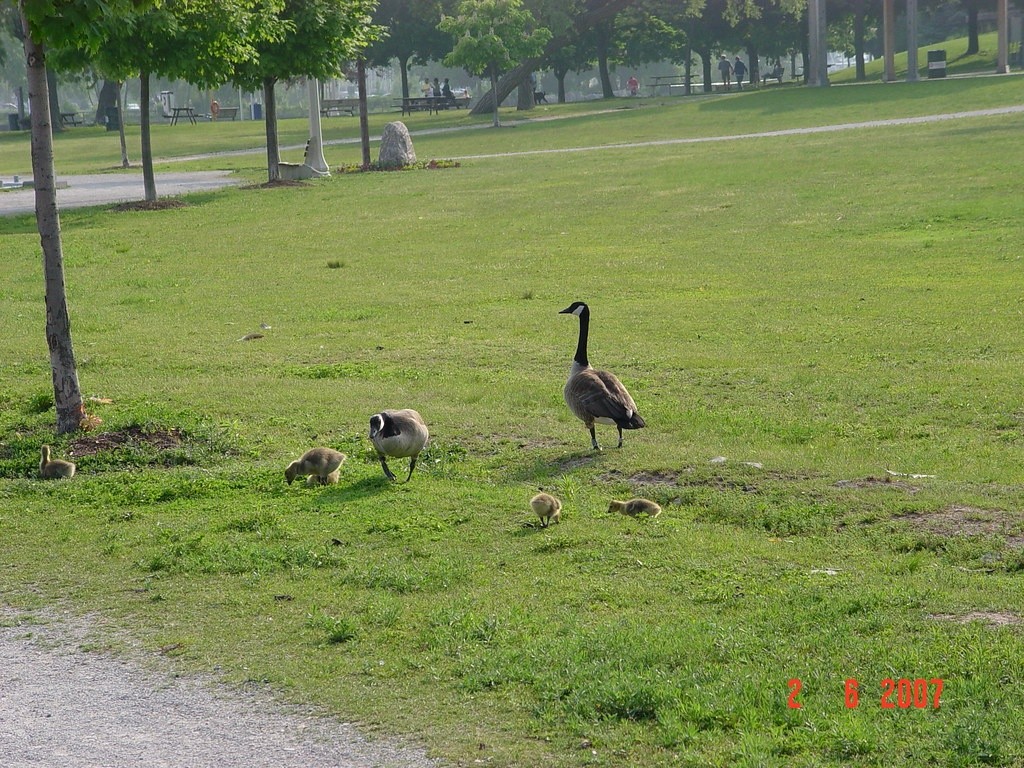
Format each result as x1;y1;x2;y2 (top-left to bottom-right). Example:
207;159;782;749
284;447;346;485
530;493;562;527
39;445;76;479
558;302;645;449
608;498;661;518
369;409;428;485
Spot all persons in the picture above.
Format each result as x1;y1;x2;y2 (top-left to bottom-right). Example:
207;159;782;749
718;55;734;90
463;90;470;109
761;60;782;85
530;72;536;92
421;78;450;110
627;76;640;96
731;56;748;89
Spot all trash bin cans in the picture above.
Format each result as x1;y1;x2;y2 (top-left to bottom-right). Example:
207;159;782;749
249;103;262;120
927;49;946;79
8;112;20;131
103;106;120;132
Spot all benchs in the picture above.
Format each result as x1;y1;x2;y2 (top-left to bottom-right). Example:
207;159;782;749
205;108;239;121
162;115;172;119
194;114;204;117
321;99;359;118
63;122;82;127
761;68;784;84
454;96;472;109
390;105;443;107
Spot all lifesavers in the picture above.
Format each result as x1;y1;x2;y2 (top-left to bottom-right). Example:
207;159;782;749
211;101;220;114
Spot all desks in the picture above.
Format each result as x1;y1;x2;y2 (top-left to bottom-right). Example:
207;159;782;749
171;108;197;127
391;96;445;117
61;112;78;121
